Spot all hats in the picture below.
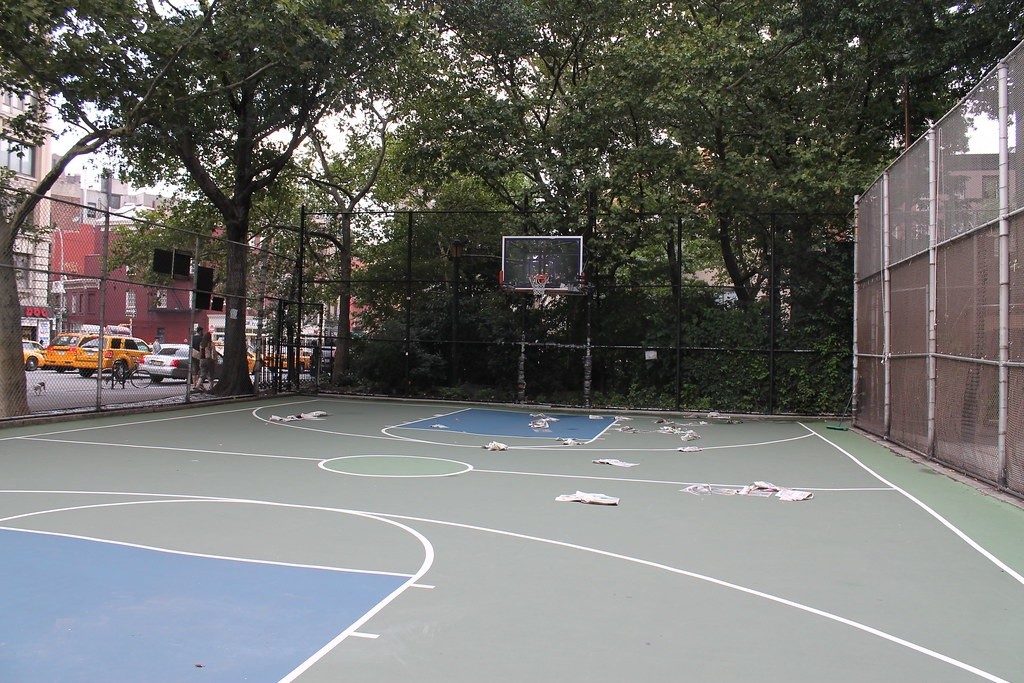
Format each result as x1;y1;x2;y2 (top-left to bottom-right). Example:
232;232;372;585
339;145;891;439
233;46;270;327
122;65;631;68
155;334;163;338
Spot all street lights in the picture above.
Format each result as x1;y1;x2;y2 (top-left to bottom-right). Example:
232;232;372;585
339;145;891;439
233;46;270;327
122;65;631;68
449;233;465;387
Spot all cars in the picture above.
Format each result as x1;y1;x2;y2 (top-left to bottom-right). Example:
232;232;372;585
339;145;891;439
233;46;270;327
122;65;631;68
73;334;154;377
145;346;223;383
22;333;87;373
214;341;355;376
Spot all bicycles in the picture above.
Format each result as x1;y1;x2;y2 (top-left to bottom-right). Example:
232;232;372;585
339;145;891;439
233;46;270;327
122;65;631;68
97;356;153;389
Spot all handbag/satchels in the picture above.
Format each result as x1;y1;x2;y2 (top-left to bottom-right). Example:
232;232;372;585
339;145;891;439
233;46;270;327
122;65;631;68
191;348;200;360
204;341;213;359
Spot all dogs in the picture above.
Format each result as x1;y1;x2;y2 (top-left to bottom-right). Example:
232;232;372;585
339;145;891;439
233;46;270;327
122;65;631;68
34;382;46;395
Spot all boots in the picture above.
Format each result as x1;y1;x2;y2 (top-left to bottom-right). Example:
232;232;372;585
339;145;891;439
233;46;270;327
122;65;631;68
194;378;206;392
208;380;214;391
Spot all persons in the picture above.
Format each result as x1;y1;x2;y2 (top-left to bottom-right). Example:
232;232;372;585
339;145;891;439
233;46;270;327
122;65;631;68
153;337;162;355
190;327;219;391
39;340;46;348
310;340;323;378
